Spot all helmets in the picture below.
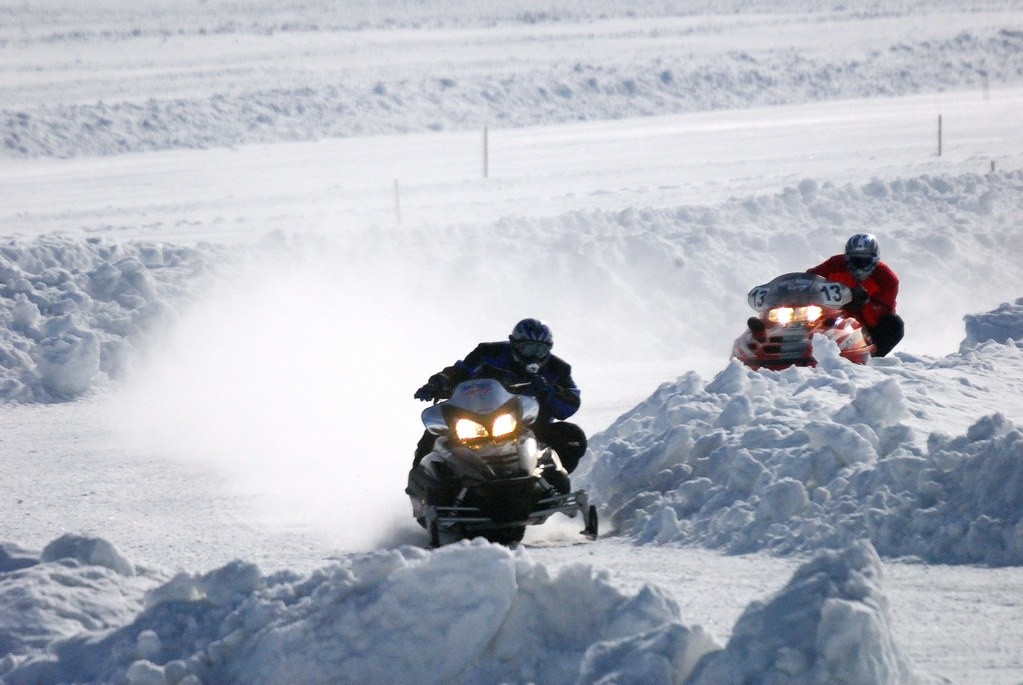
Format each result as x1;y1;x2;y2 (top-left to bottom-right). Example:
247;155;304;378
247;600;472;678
510;318;551;372
845;234;877;281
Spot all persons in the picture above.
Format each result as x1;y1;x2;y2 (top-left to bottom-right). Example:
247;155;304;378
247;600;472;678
749;233;904;357
405;319;588;498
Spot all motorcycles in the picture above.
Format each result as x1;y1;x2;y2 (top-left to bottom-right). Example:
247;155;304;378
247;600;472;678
730;272;877;372
405;381;598;547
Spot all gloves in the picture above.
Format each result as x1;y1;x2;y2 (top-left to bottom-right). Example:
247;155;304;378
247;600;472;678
531;374;548;394
416;382;444;405
850;286;868;306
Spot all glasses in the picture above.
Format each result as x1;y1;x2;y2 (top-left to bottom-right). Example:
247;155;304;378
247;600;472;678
514;342;550;358
848;254;874;270
